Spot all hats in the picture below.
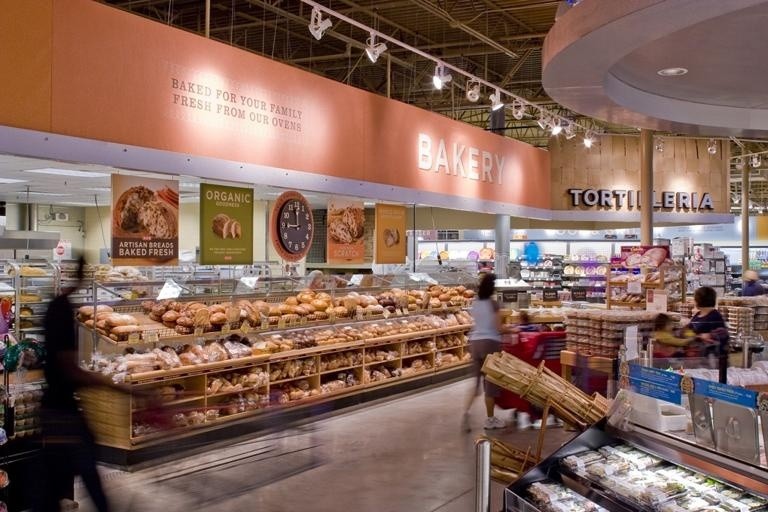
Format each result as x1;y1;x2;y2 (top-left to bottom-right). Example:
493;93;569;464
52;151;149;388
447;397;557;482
743;271;759;281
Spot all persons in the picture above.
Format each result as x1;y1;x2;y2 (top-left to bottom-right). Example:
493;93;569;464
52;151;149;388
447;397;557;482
680;287;729;363
478;261;495;282
32;250;110;512
460;273;507;433
741;269;765;296
650;313;700;370
0;429;80;511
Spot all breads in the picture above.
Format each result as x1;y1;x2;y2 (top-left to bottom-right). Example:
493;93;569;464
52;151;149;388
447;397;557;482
8;263;477;436
329;206;365;244
212;213;242;238
114;184;178;240
625;247;668;267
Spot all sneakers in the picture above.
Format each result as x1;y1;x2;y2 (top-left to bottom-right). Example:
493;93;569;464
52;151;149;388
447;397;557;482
484;417;506;428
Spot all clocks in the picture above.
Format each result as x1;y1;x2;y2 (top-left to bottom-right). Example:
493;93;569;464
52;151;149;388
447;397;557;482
277;198;313;254
